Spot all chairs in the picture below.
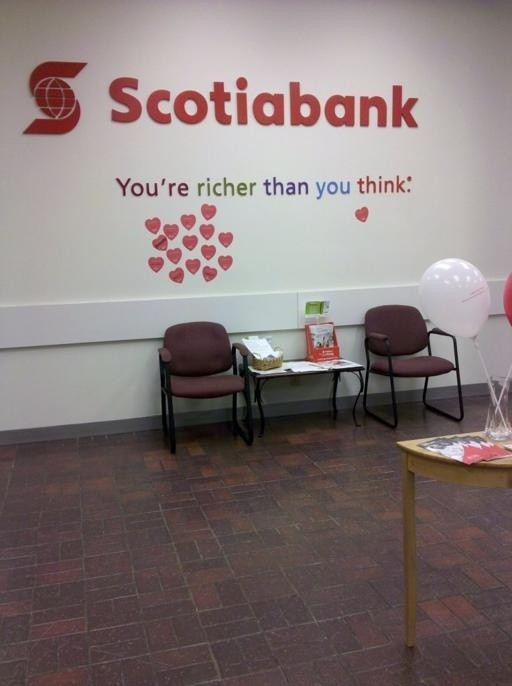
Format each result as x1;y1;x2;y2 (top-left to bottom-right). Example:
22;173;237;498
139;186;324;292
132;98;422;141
157;321;255;455
364;303;465;431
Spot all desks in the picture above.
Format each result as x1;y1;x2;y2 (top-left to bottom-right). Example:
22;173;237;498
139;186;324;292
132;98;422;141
240;356;365;438
395;424;512;647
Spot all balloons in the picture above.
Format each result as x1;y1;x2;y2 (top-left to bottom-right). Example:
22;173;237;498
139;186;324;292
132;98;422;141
501;271;512;326
419;257;490;339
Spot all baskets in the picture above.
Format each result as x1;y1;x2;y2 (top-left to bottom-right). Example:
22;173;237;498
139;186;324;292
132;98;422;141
253;351;284;371
